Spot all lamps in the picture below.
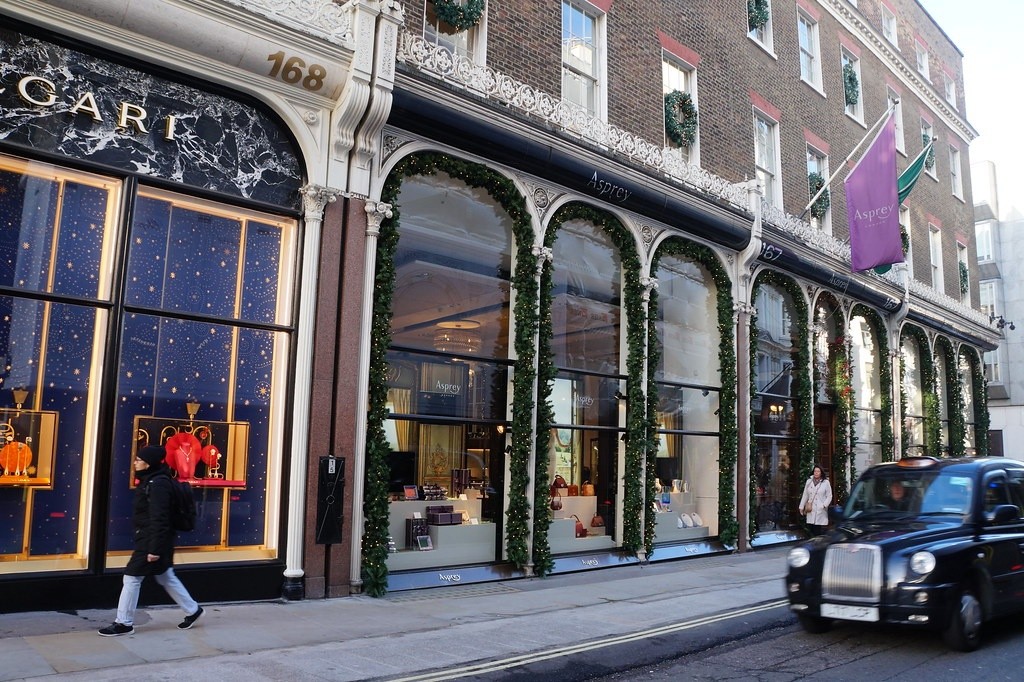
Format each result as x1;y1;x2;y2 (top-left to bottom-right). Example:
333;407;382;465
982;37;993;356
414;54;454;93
620;433;625;443
702;390;709;397
433;327;483;358
614;391;627;401
496;425;512;434
504;444;512;457
713;408;720;416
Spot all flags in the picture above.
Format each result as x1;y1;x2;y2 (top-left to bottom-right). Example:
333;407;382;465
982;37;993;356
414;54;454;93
844;107;905;271
874;146;931;276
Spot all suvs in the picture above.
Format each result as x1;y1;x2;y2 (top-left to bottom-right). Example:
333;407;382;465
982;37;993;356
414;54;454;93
784;452;1024;653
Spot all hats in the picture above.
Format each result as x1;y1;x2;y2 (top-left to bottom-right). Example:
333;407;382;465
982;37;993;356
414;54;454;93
136;445;166;465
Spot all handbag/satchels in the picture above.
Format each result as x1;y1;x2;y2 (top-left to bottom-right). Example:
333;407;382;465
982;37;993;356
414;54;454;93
553;475;568;488
581;481;595;496
571;515;583;534
591;512;604;527
550;493;562;510
805;503;812;512
568;485;578;496
549;484;556;497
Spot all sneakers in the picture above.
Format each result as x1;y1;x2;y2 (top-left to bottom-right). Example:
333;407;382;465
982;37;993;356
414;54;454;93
177;605;205;629
98;622;134;636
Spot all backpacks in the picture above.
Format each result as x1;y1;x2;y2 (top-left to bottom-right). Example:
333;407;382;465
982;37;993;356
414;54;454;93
145;475;197;532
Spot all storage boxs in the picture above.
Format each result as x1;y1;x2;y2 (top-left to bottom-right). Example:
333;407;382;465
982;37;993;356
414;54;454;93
426;505;441;514
451;512;463;525
557;487;568;497
441;505;454;513
427;513;451;524
568;485;578;496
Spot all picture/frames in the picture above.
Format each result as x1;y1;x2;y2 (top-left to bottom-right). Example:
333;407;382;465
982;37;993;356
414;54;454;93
404;486;419;499
417;536;434;550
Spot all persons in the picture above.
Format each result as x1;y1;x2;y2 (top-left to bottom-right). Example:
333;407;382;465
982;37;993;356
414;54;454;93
881;479;916;511
799;465;832;540
98;444;206;637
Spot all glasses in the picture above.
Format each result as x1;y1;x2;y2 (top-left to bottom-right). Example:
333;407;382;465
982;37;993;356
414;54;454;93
134;457;142;462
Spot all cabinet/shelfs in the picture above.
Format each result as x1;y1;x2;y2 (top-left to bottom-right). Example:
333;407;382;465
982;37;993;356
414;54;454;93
405;518;428;550
